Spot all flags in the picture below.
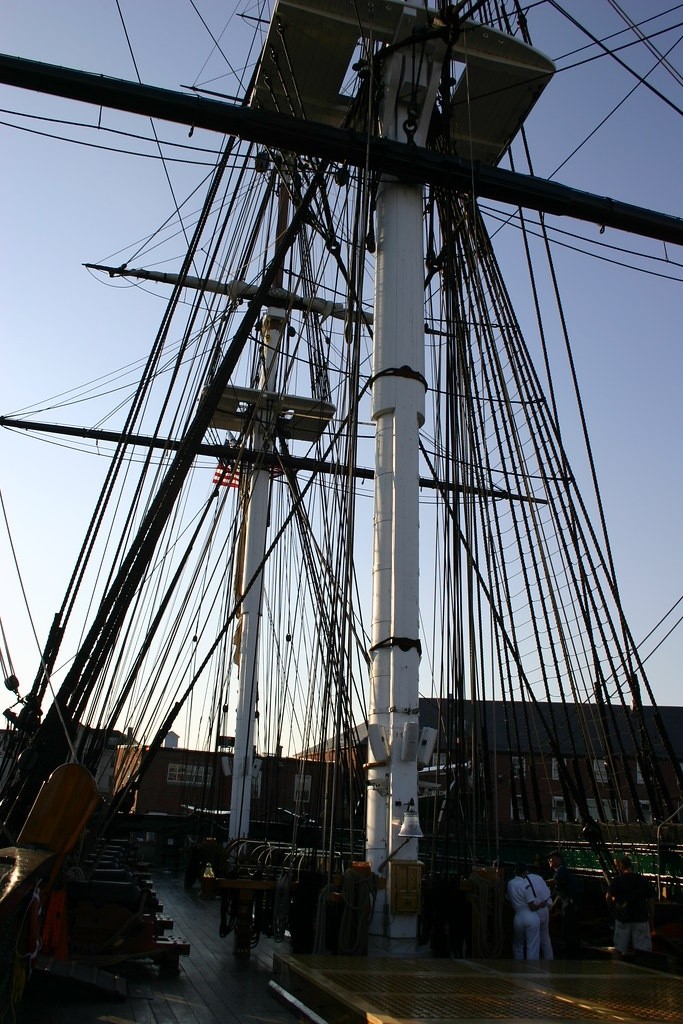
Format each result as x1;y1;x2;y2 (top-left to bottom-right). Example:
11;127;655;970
213;441;243;487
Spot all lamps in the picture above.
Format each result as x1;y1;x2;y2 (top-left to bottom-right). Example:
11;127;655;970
398;798;424;838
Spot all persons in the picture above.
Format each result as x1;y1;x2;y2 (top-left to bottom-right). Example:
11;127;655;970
507;862;555;961
544;853;587;922
605;856;656;953
